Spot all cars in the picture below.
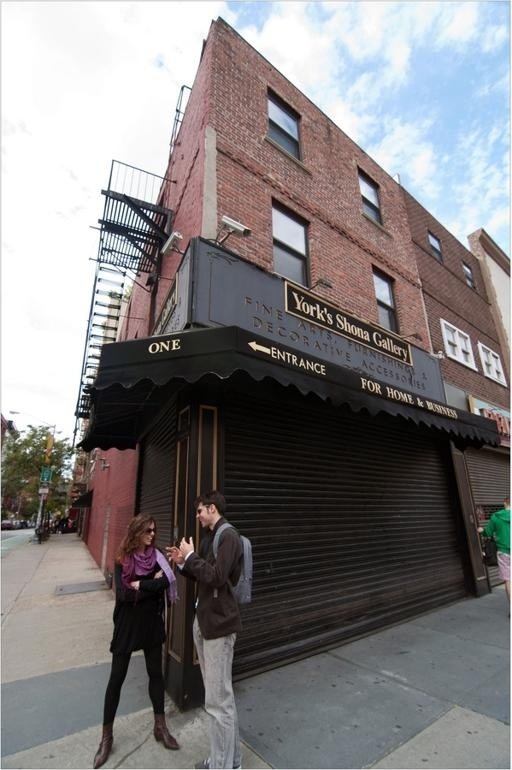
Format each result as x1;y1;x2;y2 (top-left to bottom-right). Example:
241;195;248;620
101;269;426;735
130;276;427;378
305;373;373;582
2;519;28;529
49;519;56;534
62;520;78;533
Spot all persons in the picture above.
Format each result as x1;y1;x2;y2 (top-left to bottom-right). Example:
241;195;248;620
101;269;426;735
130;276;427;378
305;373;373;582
91;515;181;769
164;490;245;769
476;497;509;617
50;516;74;534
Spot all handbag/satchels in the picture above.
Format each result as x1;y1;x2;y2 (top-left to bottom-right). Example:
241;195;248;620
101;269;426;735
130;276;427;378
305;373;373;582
484;540;498;566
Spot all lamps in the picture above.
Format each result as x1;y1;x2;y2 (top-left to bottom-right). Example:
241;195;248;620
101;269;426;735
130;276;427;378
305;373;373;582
308;278;332;292
145;273;173;286
400;333;423;342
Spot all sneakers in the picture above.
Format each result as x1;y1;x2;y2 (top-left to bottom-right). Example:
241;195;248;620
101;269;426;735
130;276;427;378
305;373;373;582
194;758;243;769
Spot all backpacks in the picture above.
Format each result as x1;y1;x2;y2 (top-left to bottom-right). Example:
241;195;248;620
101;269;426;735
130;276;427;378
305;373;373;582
213;523;254;604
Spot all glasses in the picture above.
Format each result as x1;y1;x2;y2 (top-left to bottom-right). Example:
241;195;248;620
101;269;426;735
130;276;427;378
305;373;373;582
198;504;210;514
145;527;155;534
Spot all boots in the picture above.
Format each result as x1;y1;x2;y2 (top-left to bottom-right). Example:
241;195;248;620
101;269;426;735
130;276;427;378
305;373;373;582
94;722;113;768
154;712;179;750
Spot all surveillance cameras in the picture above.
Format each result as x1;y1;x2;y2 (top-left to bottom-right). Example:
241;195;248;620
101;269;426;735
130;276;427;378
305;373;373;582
160;231;184;256
221;215;252;238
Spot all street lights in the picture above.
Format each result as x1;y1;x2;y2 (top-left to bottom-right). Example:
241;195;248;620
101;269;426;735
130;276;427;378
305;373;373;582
10;410;57;532
